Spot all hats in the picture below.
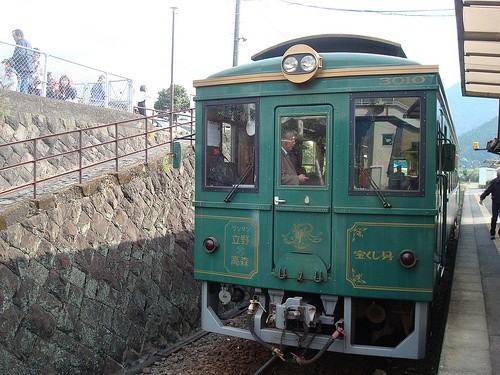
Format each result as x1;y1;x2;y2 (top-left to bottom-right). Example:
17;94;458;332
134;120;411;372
495;167;500;174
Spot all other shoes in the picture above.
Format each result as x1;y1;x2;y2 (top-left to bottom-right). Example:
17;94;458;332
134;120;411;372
490;235;495;240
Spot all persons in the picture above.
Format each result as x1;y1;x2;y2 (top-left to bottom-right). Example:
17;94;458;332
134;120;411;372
393;165;405;178
409;168;416;176
280;126;310;184
479;168;500;240
28;48;44;96
0;58;78;100
137;84;151;116
90;74;106;103
8;29;34;93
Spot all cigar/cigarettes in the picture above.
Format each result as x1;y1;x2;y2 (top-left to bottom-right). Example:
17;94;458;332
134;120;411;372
304;177;308;179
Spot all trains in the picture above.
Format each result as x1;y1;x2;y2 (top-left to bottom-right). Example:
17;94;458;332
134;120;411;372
171;34;460;367
